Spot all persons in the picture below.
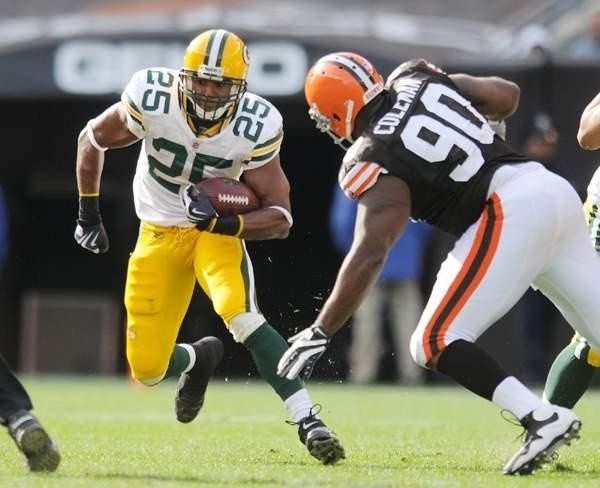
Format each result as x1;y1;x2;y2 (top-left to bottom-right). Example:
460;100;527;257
332;185;432;386
517;114;574;385
1;193;61;472
278;52;600;478
77;28;346;466
543;91;600;409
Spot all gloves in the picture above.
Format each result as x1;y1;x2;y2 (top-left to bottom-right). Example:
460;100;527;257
181;185;218;232
487;118;506;142
275;322;331;382
72;196;109;254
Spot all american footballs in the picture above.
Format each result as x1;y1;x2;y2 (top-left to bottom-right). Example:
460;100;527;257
191;178;261;217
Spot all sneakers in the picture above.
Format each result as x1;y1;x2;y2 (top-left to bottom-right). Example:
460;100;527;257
172;336;224;422
7;410;61;472
297;417;345;466
503;404;582;477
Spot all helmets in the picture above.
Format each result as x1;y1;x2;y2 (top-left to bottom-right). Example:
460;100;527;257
304;51;385;139
179;30;249;125
